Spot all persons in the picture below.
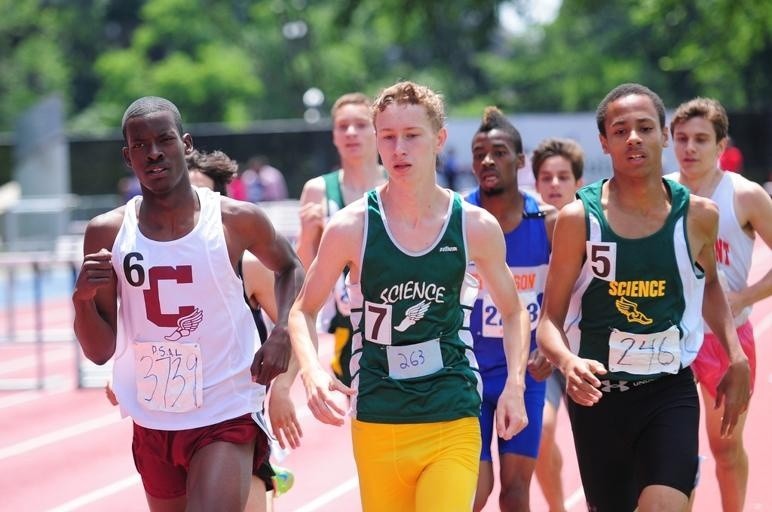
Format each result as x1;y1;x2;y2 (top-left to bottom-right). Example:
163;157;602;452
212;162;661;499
718;135;744;175
662;97;772;511
444;148;461;192
463;105;559;511
529;137;584;511
73;96;306;511
288;82;531;511
535;84;750;511
294;92;388;406
